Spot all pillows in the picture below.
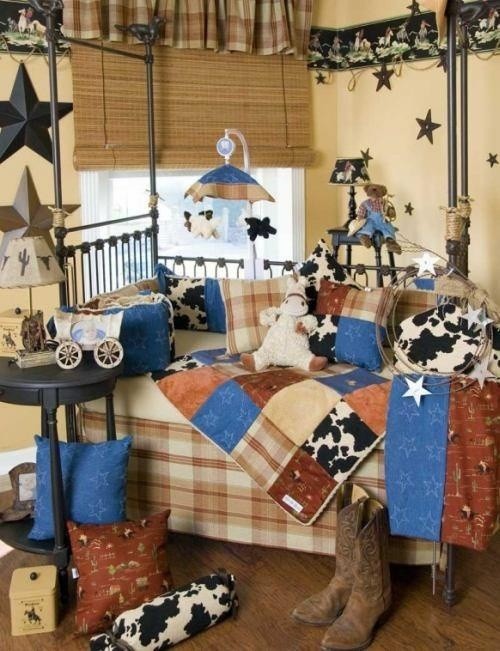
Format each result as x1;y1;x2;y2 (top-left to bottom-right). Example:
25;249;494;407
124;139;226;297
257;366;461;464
304;275;392;368
158;267;230;336
218;276;304;353
56;510;174;638
91;572;246;651
72;302;174;374
28;435;131;544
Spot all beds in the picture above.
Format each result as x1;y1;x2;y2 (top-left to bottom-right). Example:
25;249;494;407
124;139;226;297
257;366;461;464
32;210;486;626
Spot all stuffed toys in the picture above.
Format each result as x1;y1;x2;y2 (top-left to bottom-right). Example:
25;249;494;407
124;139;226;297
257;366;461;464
182;138;276;242
240;276;327;371
358;183;403;255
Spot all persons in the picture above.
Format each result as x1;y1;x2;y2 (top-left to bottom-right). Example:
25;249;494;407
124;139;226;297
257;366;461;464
78;321;100;345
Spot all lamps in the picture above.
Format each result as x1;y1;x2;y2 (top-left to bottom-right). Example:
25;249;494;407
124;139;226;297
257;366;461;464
0;236;67;368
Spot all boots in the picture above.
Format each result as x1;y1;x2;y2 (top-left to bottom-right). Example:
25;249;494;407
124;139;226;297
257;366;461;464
321;498;396;651
289;483;370;628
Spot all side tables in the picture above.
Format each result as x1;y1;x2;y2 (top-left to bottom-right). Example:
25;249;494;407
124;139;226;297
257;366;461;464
0;352;125;607
